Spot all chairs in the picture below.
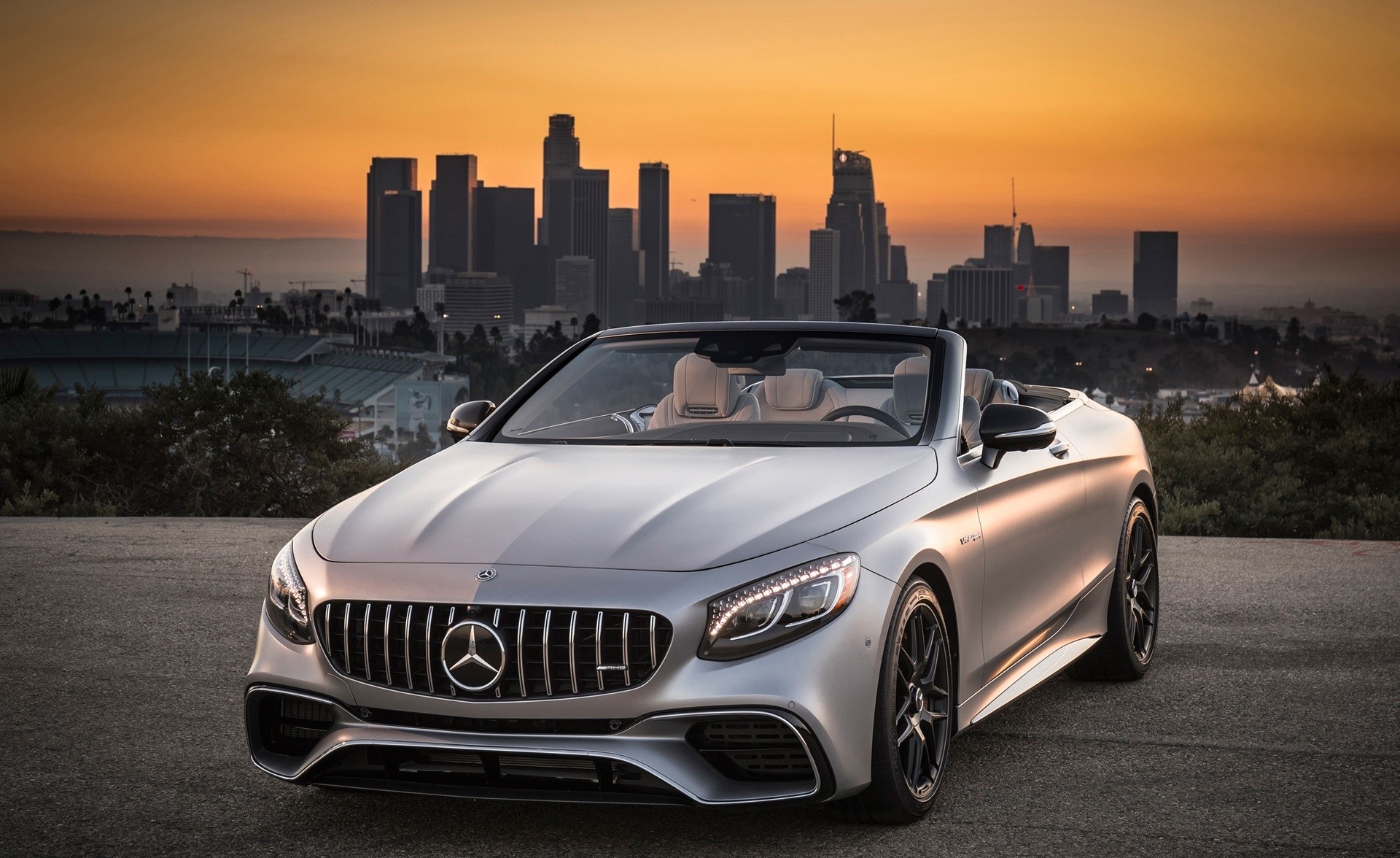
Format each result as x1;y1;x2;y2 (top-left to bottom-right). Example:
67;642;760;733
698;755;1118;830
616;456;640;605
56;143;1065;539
646;353;1018;451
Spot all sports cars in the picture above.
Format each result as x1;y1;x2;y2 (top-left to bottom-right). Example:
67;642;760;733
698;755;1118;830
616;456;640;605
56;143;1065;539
243;319;1162;828
